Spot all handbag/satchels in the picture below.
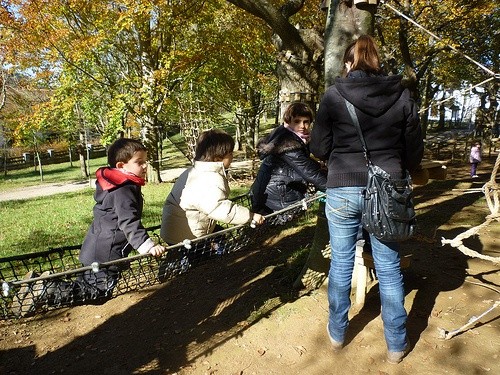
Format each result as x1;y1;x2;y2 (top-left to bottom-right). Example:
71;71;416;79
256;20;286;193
362;161;417;242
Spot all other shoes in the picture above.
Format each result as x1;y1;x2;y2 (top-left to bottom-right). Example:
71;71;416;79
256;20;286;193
386;336;411;364
12;270;41;316
21;271;60;316
327;323;344;349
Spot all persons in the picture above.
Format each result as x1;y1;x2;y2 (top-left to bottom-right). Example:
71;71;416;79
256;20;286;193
157;130;266;283
309;35;424;364
250;101;329;241
469;142;482;179
12;138;166;318
